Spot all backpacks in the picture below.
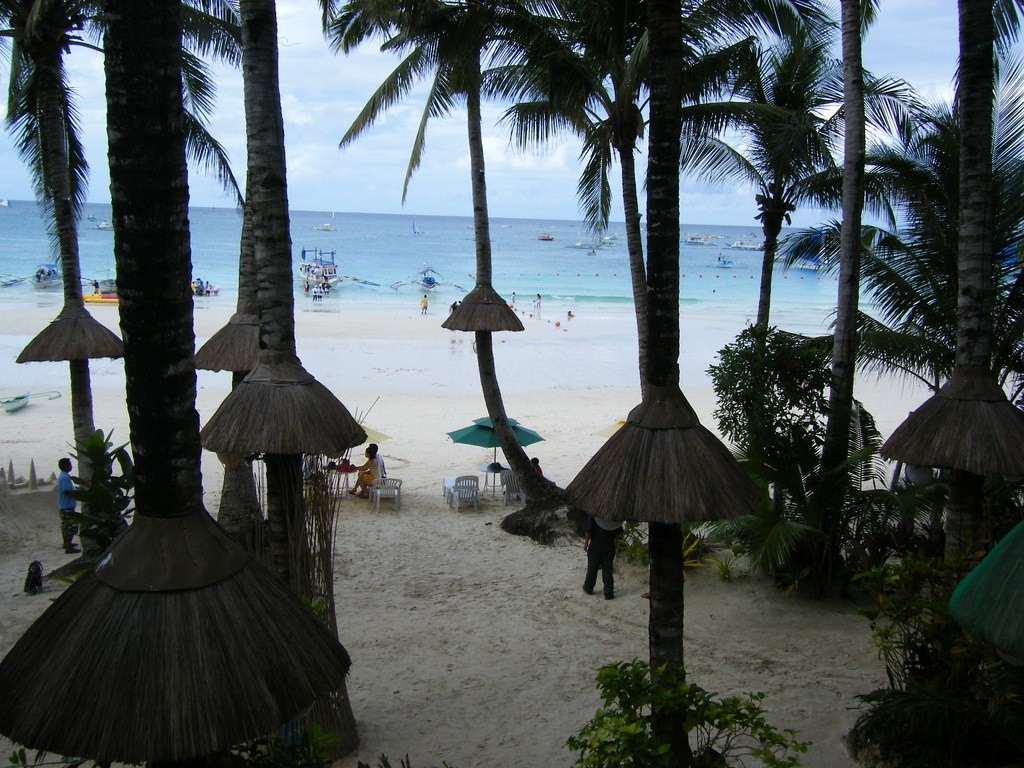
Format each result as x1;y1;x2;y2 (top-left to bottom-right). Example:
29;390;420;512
24;560;43;595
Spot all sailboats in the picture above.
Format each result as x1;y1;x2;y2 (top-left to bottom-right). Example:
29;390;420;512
413;214;423;235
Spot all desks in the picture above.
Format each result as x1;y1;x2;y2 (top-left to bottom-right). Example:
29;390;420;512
323;466;360;496
478;463;511;495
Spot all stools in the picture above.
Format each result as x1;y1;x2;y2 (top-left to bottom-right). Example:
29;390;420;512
367;485;380;509
442;477;459;495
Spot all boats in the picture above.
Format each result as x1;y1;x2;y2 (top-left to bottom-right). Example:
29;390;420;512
685;231;787;268
0;391;30;415
311;223;341;231
28;275;61;288
297;247;339;286
416;276;438;291
83;291;119;305
538;235;554;241
567;232;622;256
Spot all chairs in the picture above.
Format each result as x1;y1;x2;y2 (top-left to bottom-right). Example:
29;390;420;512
372;478;403;513
446;475;479;512
499;470;525;506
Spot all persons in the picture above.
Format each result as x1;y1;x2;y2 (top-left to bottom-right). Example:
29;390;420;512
450;301;461;313
568;311;574;316
536;294;541;306
348;444;386;498
511;292;515;303
57;458;81;553
191;281;212;296
421;295;428;314
302;264;330;301
36;269;55;281
583;516;624;600
93;280;100;293
531;458;542;474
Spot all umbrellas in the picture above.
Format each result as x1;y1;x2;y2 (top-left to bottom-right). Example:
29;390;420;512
358;426;393;443
596;419;626;437
447;417;546;494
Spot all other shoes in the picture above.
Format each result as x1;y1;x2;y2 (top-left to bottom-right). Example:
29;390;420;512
63;544;78;548
605;593;614;600
65;548;81;554
583;585;592;595
359;495;370;499
642;592;651;598
347;490;356;496
